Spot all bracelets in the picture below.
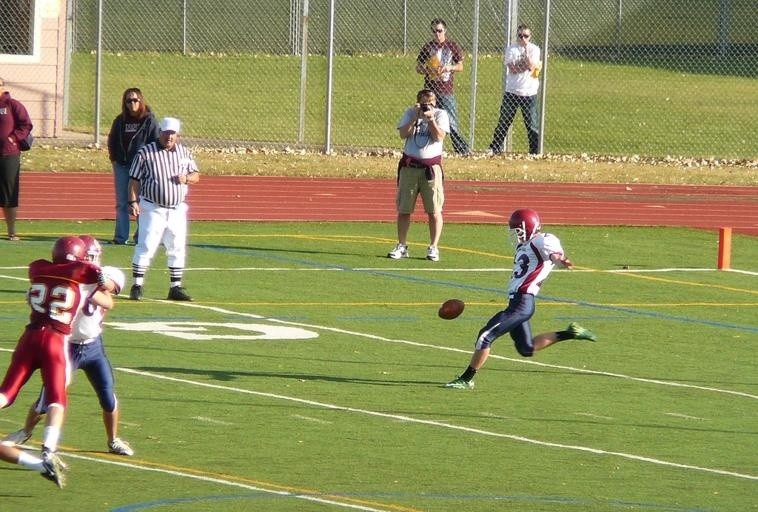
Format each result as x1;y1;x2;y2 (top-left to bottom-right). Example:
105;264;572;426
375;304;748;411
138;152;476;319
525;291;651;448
427;116;437;122
127;200;138;206
185;174;190;184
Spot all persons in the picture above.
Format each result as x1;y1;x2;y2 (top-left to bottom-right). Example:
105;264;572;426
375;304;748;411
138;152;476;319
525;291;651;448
483;23;543;159
386;89;450;262
7;235;134;456
0;235;114;490
108;88;161;246
416;18;471;157
0;444;67;489
442;207;599;389
127;115;199;303
0;77;33;241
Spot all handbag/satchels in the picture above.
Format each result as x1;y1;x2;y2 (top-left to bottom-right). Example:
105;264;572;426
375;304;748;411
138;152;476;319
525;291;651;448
17;134;32;150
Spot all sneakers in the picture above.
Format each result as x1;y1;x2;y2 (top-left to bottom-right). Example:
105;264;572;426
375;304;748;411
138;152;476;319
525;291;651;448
39;459;65;489
387;243;409;259
167;286;194;301
444;377;473;389
0;429;32;446
566;323;597;343
39;444;66;471
9;232;19;240
427;246;439;261
107;438;134;455
129;284;143;300
108;240;127;244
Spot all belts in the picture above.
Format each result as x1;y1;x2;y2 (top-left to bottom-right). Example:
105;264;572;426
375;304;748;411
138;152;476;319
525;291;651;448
144;198;175;209
405;162;426;170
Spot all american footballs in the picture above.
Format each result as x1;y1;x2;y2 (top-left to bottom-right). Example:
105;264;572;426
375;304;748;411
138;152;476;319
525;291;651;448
438;299;464;318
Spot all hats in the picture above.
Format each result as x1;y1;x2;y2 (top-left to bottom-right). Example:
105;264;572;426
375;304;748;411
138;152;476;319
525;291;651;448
158;117;179;132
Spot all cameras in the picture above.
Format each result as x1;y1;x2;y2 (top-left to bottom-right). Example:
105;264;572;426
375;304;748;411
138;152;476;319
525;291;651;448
418;103;433;119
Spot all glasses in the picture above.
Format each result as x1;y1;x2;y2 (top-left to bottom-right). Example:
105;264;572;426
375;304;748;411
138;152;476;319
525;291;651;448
125;98;138;103
430;28;443;32
0;84;3;87
416;89;433;96
518;33;529;38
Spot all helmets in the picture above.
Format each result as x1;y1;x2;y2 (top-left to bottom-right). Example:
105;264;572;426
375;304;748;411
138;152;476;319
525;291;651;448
507;209;540;248
79;235;102;267
51;236;88;263
